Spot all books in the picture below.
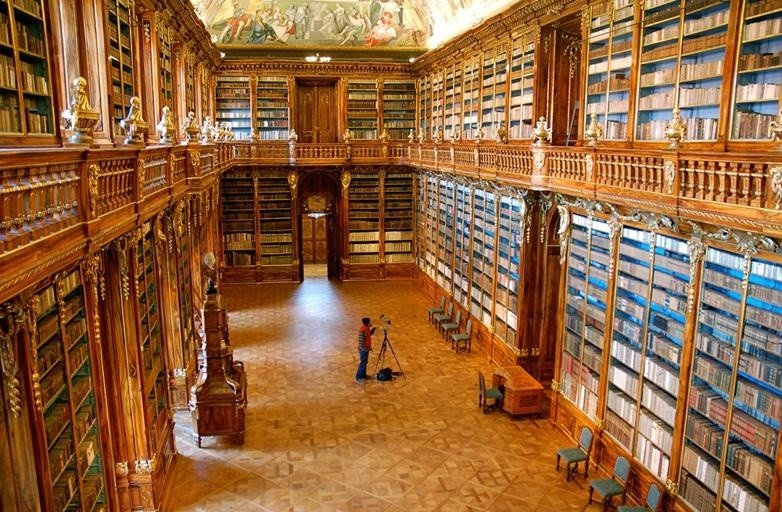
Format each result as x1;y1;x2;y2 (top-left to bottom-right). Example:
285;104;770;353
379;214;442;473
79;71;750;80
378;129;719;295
348;173;412;263
559;212;782;512
176;233;195;363
588;0;782;138
347;82;416;139
222;178;293;264
30;270;106;511
0;0;51;135
416;172;523;348
106;1;135;137
216;82;287;140
421;44;534;140
158;39;208;125
135;238;168;448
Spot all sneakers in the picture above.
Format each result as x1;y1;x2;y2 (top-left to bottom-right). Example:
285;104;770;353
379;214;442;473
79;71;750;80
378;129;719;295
355;375;372;382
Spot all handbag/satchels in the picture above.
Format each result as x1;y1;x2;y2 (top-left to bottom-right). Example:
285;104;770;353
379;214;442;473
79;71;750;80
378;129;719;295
376;367;393;382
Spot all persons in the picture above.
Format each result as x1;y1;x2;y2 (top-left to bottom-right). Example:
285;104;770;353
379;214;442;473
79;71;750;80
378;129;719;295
355;318;376;382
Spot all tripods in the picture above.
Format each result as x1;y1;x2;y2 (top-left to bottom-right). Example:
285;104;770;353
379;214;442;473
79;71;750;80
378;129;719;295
370;330;406;382
